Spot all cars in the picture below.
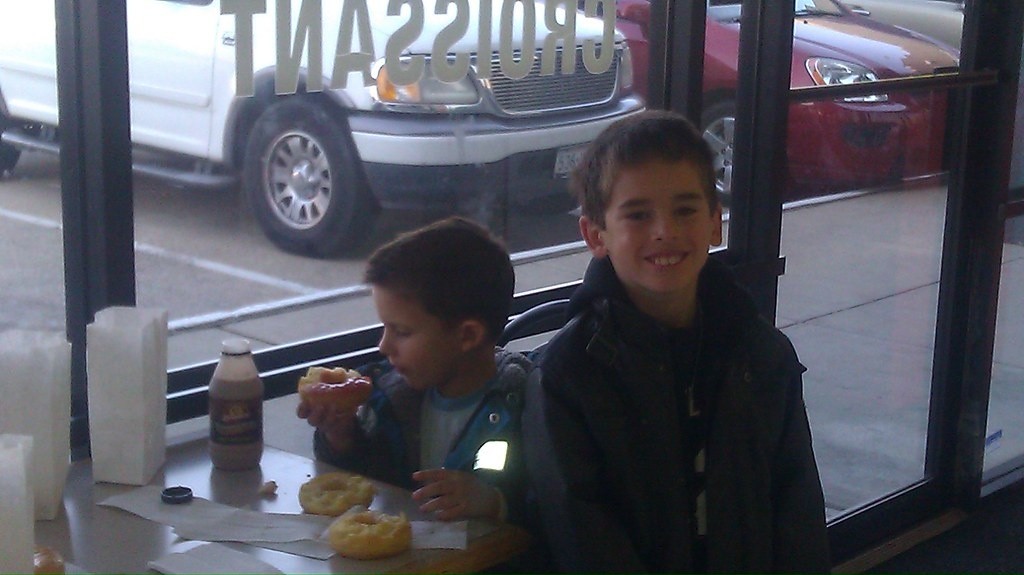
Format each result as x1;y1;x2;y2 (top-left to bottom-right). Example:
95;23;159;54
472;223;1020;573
0;0;645;261
576;0;968;194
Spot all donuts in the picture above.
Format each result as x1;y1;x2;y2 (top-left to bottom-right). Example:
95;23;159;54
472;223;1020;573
32;544;66;575
328;510;412;560
299;471;376;517
298;366;373;409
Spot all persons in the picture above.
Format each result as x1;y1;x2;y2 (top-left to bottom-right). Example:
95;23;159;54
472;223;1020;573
297;217;538;521
522;112;827;575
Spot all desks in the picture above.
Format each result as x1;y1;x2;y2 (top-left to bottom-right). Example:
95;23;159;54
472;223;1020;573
34;428;534;575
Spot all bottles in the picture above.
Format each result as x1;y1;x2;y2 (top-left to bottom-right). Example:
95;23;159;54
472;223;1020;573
209;338;264;470
